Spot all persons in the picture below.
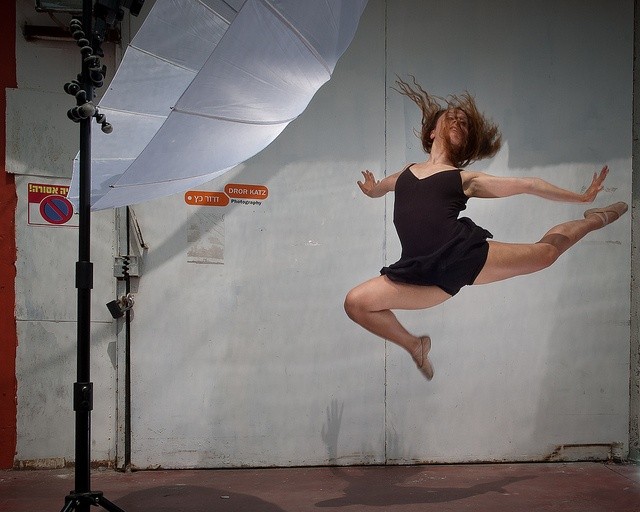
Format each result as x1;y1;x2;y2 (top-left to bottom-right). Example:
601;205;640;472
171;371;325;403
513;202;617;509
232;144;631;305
344;72;628;381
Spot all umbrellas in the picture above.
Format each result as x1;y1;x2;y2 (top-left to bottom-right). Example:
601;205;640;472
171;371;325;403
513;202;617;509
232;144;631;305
64;0;368;215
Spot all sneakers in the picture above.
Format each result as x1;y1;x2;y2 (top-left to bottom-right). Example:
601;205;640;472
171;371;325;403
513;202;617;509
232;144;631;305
412;336;433;380
584;202;628;227
